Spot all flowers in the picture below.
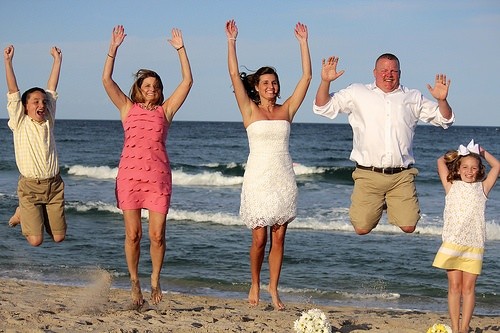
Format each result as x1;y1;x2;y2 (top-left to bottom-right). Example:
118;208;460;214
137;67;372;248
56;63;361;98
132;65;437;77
293;309;333;333
425;323;452;333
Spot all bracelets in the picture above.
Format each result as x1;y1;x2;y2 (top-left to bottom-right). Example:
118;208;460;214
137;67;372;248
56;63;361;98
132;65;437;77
229;38;236;41
108;54;114;59
177;45;184;51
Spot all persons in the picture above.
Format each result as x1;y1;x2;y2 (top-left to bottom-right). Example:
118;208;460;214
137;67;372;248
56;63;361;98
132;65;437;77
315;53;454;237
5;44;66;247
103;25;193;304
432;138;500;333
225;19;312;309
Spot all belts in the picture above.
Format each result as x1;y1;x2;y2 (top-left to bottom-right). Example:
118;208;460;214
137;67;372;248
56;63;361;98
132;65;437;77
357;163;410;175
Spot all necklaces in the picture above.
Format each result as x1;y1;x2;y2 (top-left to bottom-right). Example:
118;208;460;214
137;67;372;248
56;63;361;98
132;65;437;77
259;102;274;107
